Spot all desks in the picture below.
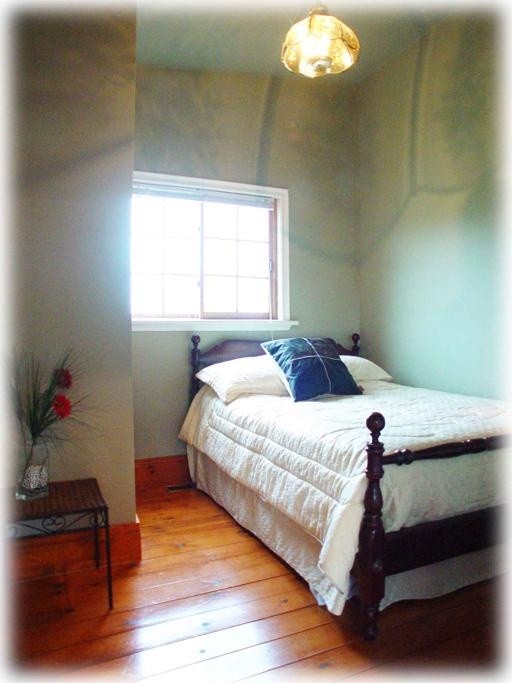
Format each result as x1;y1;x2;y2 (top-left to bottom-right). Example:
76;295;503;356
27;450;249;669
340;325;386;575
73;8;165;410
16;475;114;613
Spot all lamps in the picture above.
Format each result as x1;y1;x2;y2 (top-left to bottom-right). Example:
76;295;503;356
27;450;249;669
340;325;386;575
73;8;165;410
277;1;361;79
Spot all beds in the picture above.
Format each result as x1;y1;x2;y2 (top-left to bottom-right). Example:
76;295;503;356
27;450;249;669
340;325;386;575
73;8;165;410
184;327;508;638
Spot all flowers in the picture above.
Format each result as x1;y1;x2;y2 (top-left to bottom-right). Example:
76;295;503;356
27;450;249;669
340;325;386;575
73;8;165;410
10;345;101;473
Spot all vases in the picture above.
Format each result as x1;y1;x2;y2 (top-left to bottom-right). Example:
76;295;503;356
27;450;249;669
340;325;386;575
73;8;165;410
16;438;51;501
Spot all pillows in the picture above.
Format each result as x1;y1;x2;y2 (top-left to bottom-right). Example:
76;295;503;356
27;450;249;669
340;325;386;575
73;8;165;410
195;334;392;407
